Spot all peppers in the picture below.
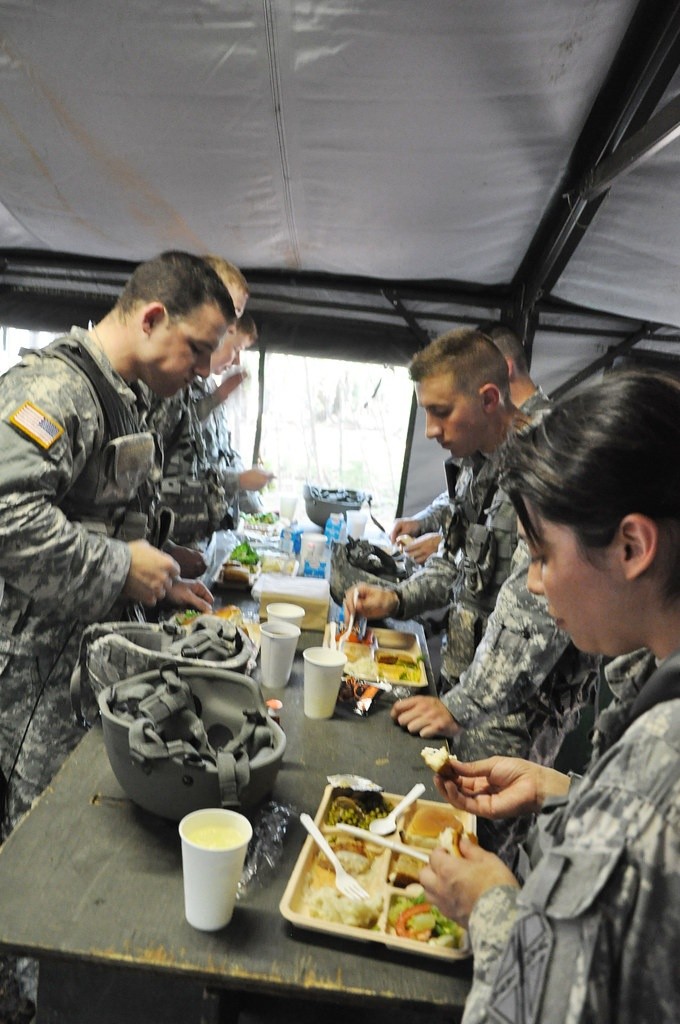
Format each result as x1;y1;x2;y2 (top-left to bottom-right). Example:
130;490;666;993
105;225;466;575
395;903;429;939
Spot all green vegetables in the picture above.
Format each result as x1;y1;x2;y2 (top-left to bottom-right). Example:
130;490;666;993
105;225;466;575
239;511;278;524
230;540;261;565
388;894;457;935
175;610;202;625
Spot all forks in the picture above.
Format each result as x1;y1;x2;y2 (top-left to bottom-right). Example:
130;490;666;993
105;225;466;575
338;588;360;653
299;813;369;903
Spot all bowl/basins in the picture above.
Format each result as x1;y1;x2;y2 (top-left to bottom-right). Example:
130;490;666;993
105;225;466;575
173;613;263;677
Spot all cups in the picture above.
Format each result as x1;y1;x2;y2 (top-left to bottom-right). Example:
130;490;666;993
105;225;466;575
279;495;298;522
179;808;253;932
346;510;367;541
258;621;301;690
302;646;348;720
300;533;328;568
266;602;305;630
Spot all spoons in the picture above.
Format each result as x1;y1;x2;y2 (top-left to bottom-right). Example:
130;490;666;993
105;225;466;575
369;783;426;836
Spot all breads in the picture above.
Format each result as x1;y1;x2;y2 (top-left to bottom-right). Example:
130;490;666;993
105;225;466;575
212;604;249;638
223;566;250;584
438;827;478;861
399;806;463;851
421;745;460;782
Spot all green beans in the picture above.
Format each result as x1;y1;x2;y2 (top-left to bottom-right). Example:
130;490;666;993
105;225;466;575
328;799;393;831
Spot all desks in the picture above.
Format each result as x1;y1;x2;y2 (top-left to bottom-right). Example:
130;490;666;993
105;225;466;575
1;526;476;1024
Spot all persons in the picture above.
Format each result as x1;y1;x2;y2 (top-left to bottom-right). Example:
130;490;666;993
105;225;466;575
420;376;680;1024
0;249;237;1024
341;326;602;871
150;258;269;580
389;320;552;564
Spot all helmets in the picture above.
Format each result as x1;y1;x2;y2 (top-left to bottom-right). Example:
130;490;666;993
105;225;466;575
85;614;251;707
304;484;372;529
329;538;407;607
97;668;287;821
